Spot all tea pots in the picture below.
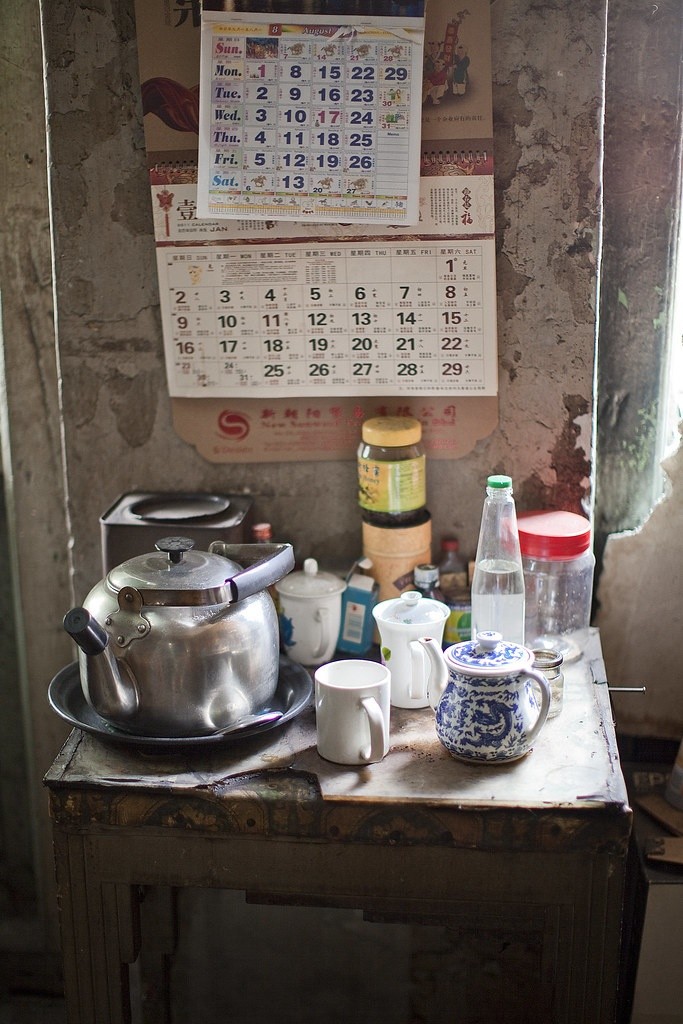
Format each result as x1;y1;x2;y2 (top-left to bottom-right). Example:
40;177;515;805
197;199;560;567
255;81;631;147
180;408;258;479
419;632;552;764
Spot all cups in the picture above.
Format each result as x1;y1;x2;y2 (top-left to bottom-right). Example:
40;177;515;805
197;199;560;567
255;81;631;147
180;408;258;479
372;592;451;708
274;558;346;666
315;659;390;766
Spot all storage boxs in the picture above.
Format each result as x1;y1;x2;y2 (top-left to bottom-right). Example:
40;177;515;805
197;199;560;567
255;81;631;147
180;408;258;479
338;558;380;653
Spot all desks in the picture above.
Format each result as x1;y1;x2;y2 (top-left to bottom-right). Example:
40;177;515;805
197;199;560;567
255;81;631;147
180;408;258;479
42;624;636;1024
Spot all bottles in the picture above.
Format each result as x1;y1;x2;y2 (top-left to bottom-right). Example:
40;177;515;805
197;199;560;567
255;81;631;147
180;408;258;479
470;475;526;645
515;509;587;659
358;415;425;525
440;540;468;590
528;647;565;721
251;522;274;544
413;566;446;604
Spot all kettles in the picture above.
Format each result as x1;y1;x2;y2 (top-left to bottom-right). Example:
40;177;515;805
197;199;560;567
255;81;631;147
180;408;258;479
60;536;295;736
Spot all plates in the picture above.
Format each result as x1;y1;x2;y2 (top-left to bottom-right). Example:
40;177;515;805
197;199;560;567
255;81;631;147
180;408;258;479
47;654;314;744
